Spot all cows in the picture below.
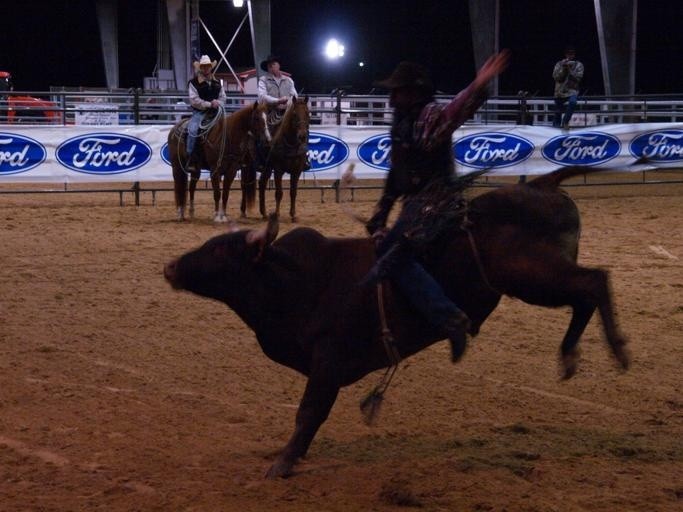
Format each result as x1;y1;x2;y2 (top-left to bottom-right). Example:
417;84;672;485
163;156;660;482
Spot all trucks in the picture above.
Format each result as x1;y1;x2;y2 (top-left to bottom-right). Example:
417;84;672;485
0;71;63;124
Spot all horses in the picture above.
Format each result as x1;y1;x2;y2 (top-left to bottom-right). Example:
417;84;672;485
167;98;274;224
238;92;310;224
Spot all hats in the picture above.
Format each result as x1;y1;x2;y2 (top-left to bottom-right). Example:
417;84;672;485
260;55;283;72
192;55;217;72
371;61;434;91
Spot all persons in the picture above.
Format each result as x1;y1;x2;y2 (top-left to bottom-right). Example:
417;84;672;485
253;52;298;171
551;42;585;133
188;55;227;172
369;42;516;364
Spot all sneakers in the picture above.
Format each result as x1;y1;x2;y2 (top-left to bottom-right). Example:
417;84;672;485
442;308;472;363
